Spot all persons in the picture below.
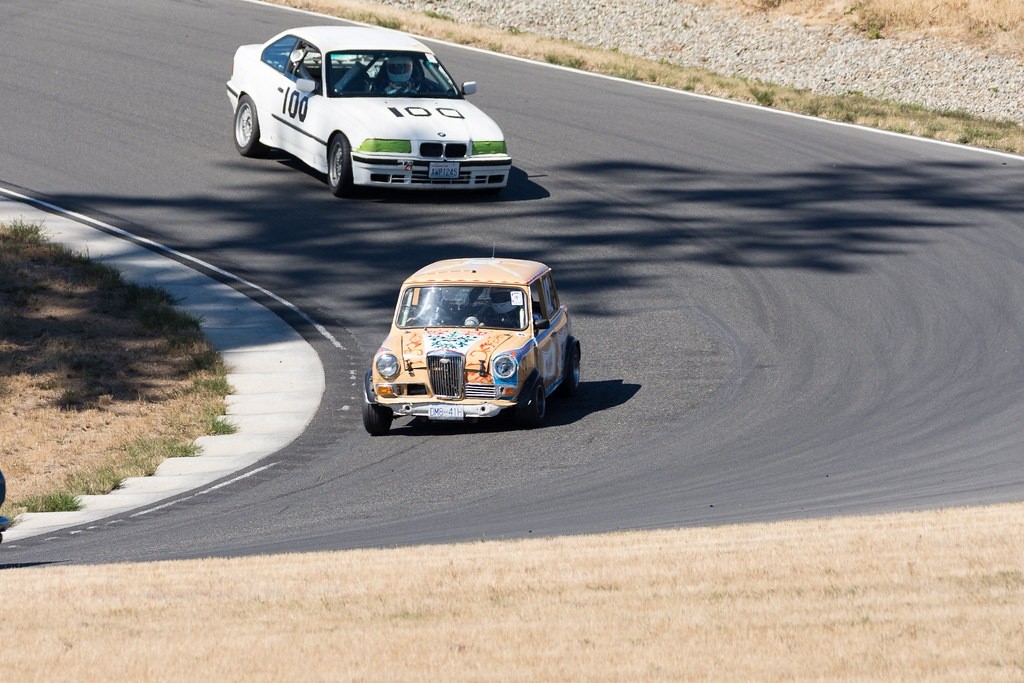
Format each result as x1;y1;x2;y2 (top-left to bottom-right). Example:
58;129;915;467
479;287;542;329
385;56;423;94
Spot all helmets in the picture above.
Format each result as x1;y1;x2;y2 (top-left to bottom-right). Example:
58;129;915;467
385;55;413;83
489;286;518;314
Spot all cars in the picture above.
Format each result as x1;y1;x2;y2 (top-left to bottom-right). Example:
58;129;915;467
361;259;582;436
226;25;512;198
